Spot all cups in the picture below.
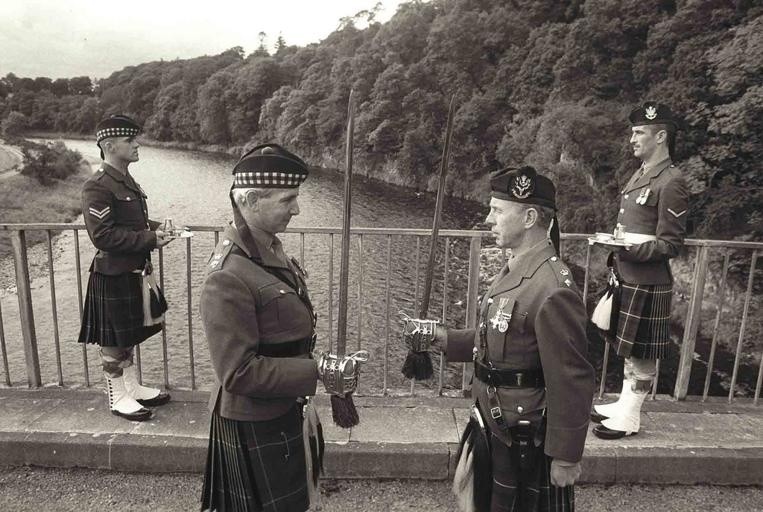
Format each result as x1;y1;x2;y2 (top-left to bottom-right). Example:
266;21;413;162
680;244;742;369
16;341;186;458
615;225;627;240
163;219;174;237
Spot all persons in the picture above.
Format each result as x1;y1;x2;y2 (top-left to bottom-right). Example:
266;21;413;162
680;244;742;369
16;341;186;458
401;166;597;510
588;101;689;440
72;114;172;421
192;142;369;510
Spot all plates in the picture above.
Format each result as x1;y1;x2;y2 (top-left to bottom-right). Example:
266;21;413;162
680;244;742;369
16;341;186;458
587;237;633;247
155;229;194;239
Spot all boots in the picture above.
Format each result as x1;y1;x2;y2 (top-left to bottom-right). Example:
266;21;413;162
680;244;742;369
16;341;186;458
107;363;170;421
591;358;656;439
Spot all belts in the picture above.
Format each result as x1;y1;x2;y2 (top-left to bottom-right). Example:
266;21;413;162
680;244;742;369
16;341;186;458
473;355;545;388
259;329;318;358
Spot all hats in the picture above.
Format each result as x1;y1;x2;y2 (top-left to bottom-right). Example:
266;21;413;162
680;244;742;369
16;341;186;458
232;143;308;188
490;164;556;211
96;115;141;144
629;100;679;129
